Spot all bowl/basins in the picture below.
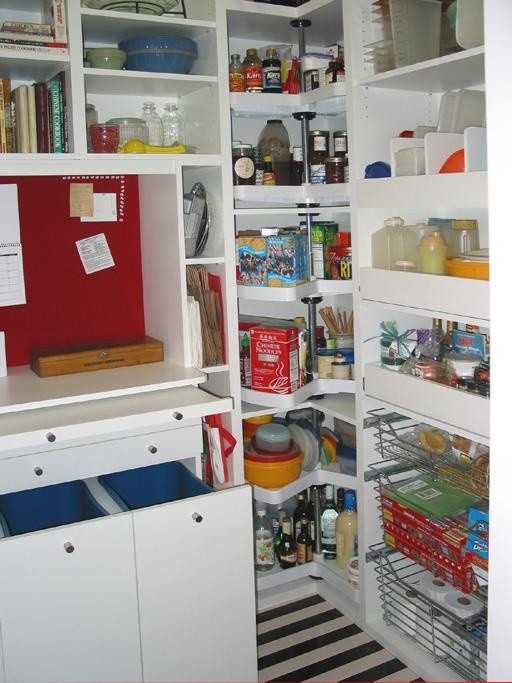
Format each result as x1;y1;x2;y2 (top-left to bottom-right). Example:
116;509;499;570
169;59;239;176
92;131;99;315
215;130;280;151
118;36;199;74
108;117;149;150
87;47;126;70
244;412;303;488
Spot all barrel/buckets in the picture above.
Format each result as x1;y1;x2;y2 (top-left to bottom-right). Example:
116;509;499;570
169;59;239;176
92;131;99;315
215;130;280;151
256;120;290;184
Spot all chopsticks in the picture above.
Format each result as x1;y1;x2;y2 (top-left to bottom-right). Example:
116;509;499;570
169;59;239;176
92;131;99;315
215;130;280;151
318;307;354;334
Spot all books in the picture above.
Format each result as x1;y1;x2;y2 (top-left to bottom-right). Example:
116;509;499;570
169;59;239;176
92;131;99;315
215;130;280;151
0;70;68;153
0;0;69;56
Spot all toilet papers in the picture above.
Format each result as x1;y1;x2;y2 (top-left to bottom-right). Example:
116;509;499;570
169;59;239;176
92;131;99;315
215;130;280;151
392;575;484;656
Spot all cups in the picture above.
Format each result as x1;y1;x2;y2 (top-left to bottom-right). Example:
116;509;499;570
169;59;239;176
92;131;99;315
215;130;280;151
90;123;121;153
395;145;426;175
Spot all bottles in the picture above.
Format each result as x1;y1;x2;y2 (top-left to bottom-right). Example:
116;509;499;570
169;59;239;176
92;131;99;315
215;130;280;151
238;332;254;388
295;315;354;386
143;101;185;152
230;47;281;92
430;317;458;361
370;215;479;275
254;484;357;571
323;55;344;85
234;117;348;185
85;104;98;153
